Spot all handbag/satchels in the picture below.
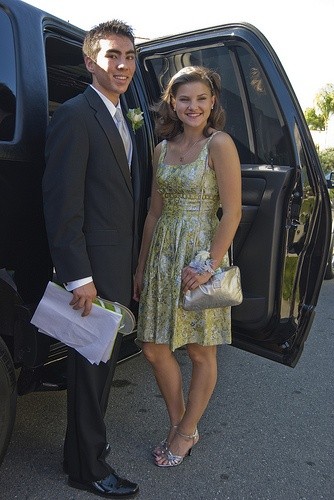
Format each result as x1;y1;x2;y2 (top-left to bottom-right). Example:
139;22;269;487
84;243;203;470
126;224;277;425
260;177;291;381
183;266;243;312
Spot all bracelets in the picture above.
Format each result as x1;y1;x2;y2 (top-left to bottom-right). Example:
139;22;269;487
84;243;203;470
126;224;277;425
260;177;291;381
188;250;216;277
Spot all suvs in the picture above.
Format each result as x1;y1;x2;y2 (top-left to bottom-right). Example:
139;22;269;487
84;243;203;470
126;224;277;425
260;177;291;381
0;0;332;476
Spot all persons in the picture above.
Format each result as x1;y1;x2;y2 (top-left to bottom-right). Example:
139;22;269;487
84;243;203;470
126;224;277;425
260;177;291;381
132;66;243;465
42;20;139;496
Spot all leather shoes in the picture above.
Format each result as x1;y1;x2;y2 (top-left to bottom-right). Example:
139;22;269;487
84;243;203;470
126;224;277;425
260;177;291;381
103;442;111;460
68;469;139;497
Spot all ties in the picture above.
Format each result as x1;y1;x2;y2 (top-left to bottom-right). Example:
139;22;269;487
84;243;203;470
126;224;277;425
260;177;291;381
113;108;132;171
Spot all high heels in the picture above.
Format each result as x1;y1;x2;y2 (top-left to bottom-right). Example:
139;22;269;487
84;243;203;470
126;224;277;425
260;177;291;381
154;424;200;468
152;425;181;457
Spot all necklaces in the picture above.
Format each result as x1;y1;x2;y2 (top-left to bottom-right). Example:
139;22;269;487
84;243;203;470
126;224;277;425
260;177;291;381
177;135;200;162
